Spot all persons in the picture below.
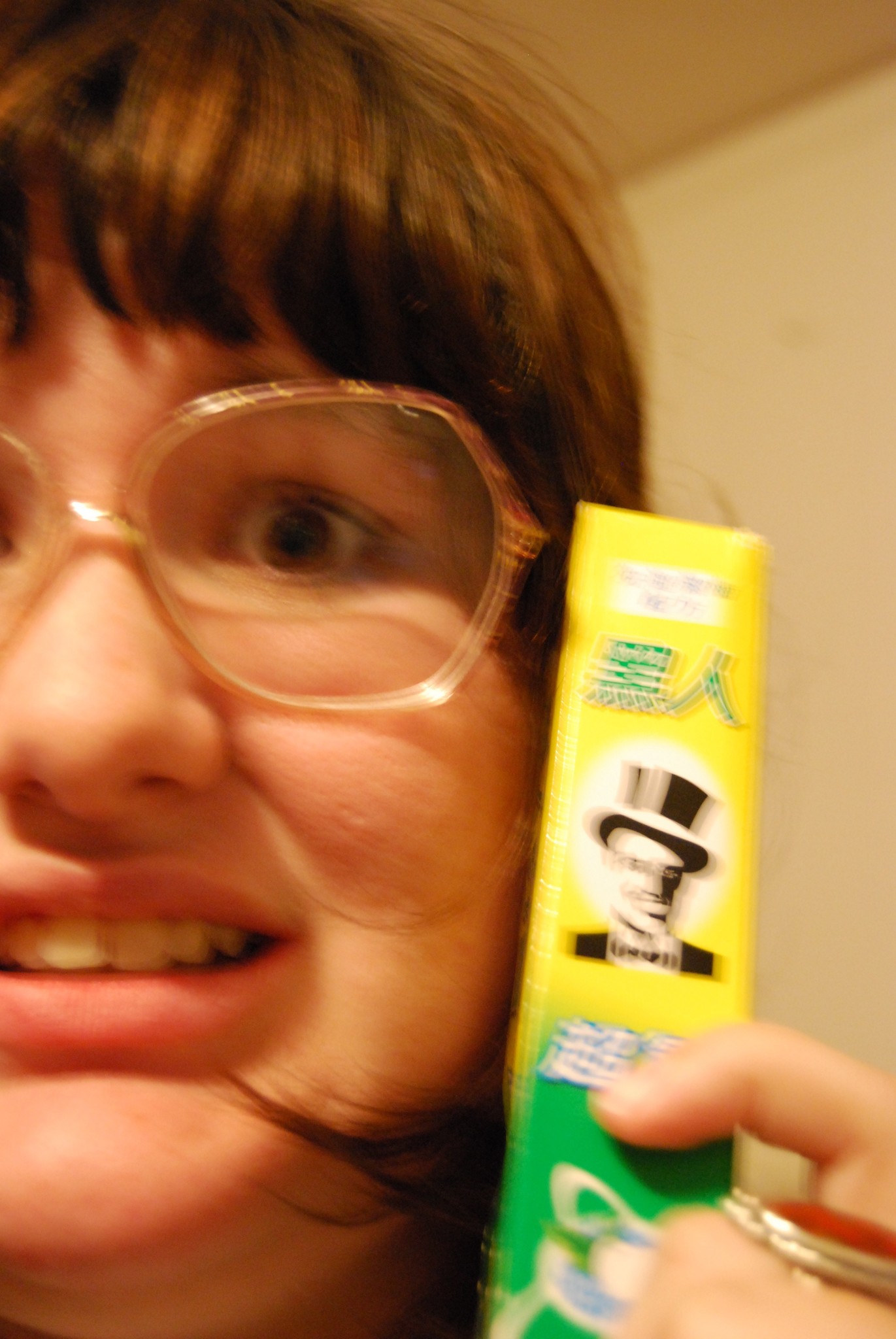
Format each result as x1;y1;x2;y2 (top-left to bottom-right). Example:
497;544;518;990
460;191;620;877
0;0;896;1339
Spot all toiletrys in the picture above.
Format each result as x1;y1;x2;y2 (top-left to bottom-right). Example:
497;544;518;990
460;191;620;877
476;505;770;1339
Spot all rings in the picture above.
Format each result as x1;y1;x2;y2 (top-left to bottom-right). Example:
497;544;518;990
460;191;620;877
722;1186;896;1297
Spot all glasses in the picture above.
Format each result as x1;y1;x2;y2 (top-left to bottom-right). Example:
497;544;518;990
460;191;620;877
0;379;551;716
